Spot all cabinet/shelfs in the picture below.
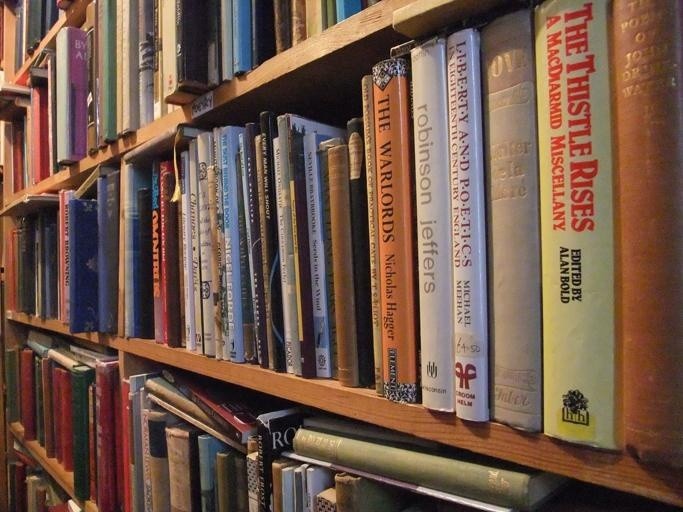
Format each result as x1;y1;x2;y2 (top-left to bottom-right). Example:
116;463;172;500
0;0;682;510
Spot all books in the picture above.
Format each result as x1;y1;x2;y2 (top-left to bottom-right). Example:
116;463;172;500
1;0;682;511
